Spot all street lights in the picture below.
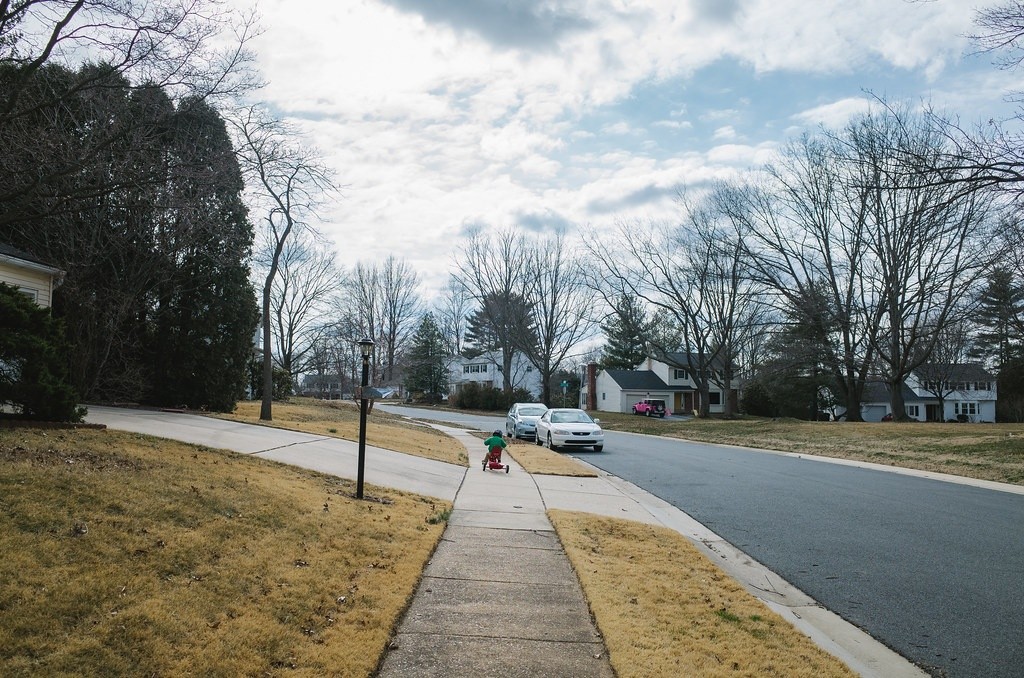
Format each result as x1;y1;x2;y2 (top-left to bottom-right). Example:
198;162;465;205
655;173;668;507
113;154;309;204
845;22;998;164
353;336;375;503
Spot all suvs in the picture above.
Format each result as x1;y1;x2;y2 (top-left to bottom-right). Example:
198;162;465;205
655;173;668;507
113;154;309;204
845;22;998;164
633;398;667;418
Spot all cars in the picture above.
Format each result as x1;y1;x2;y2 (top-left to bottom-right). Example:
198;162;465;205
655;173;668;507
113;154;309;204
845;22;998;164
507;403;548;442
535;407;605;452
881;412;914;422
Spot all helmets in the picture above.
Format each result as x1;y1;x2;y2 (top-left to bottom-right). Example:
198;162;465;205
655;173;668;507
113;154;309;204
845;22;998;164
493;430;503;438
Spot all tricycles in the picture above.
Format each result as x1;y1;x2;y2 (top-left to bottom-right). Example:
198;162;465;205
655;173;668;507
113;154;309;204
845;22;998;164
481;446;511;473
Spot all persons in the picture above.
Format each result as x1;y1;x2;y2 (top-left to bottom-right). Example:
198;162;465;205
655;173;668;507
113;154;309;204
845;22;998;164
482;430;507;462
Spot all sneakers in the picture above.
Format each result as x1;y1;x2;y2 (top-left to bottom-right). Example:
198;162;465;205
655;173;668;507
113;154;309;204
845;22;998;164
481;459;487;462
498;459;501;463
